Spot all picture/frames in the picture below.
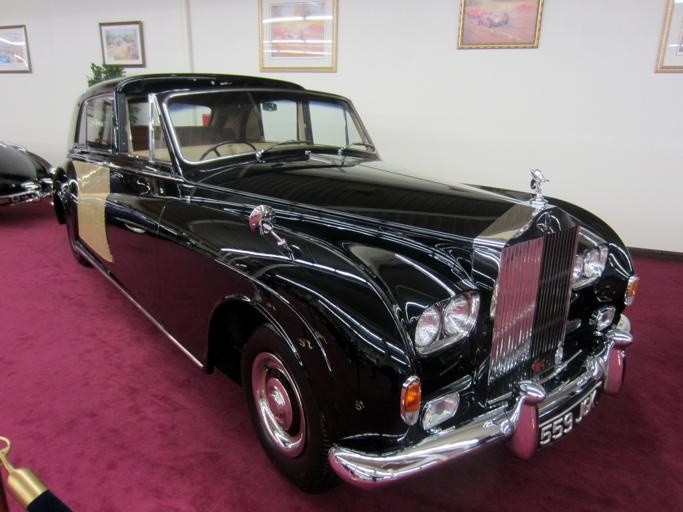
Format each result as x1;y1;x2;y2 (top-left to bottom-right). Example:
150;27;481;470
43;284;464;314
257;1;338;73
456;0;544;48
655;0;683;72
98;21;145;67
0;25;31;73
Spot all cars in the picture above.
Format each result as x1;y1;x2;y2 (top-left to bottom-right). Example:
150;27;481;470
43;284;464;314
0;140;56;209
47;73;639;496
